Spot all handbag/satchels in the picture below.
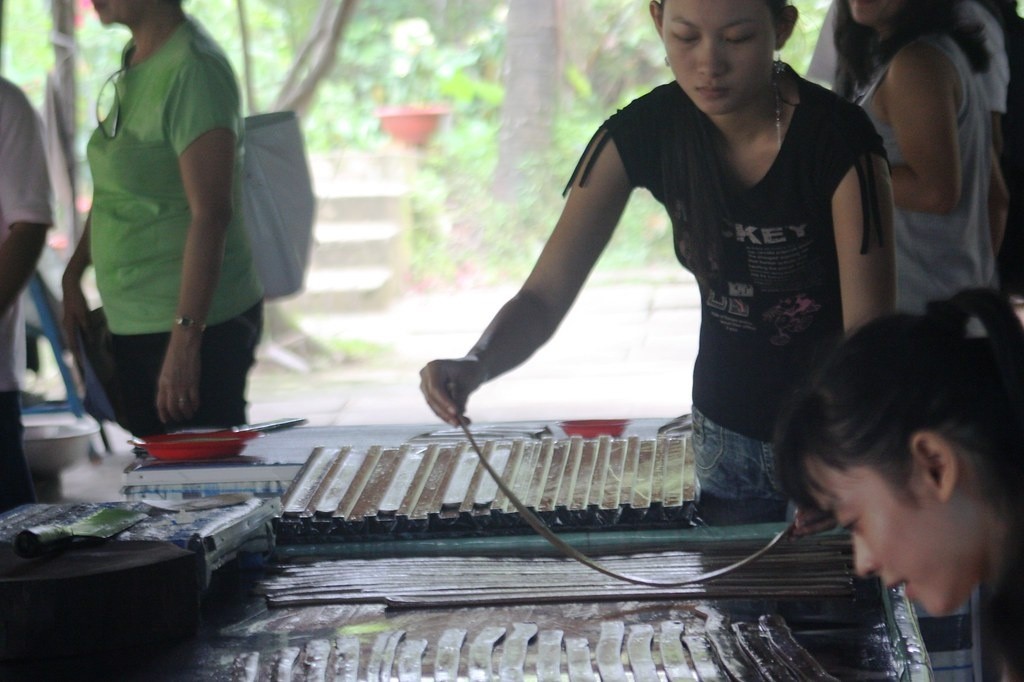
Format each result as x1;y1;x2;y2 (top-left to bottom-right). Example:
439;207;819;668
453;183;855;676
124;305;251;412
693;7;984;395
235;112;318;301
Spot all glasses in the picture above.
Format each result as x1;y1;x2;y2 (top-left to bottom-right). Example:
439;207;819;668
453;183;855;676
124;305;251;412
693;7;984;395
96;67;127;140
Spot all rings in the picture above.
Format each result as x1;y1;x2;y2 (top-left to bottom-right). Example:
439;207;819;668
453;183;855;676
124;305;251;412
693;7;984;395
178;399;190;403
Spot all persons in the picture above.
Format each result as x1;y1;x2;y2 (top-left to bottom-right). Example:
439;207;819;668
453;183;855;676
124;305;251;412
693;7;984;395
774;288;1024;682
64;0;264;438
420;0;1010;537
0;77;54;515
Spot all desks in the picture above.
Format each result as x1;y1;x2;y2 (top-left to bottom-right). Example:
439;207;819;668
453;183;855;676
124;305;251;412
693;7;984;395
0;494;283;661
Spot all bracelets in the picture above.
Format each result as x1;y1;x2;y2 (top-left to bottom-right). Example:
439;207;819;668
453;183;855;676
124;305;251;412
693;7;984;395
173;315;206;333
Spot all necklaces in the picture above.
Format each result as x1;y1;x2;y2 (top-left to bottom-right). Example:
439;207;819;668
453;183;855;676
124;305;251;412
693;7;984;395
772;77;782;152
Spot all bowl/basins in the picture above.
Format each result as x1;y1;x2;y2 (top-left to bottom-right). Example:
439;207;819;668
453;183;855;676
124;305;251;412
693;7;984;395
557;418;635;439
130;432;259;462
24;411;101;474
